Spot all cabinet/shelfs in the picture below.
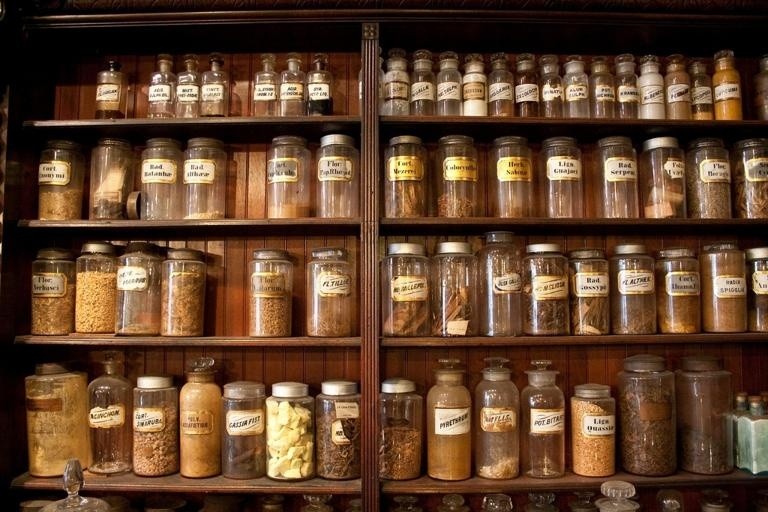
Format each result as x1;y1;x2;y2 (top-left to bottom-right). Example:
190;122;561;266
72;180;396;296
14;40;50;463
375;8;767;511
3;6;372;511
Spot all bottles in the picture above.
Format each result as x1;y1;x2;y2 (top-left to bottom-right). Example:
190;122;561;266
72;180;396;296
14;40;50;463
175;54;201;118
381;243;431;337
306;248;350;337
253;53;280;116
377;380;423;480
614;53;638;119
564;248;610;337
181;138;227;219
616;355;677;476
664;53;690;119
147;54;176;118
637;55;665;120
437;135;482;217
738;395;768;476
655;248;701;334
430;241;479;337
180;357;222;479
540;55;564;118
316;134;360;218
87;351;133;474
200;53;230;116
358;47;383;115
589;56;615;119
221;381;267;479
745;247;768;332
383;48;410;115
31;249;76;336
74;242;117;334
315;381;361;481
593;136;638;218
690;57;713;120
306;53;333;116
23;360;91;478
730;137;767;218
435;51;463;116
609;244;656;333
267;136;311;216
384;136;429;218
564;383;616;478
140;138;183;219
409;49;437;116
487;136;535;217
540;136;583;218
266;382;316;481
475;231;523;337
95;60;129;119
519;360;565;478
463;53;487;116
249;249;294;338
699;243;747;332
133;376;179;477
38;139;83;220
474;358;520;479
487;53;515;116
563;55;589;118
428;359;472;481
674;356;733;474
161;248;206;337
685;137;731;218
280;52;307;116
89;137;135;220
712;50;743;120
731;392;748;467
638;137;686;218
523;243;570;335
514;53;540;117
115;242;167;336
